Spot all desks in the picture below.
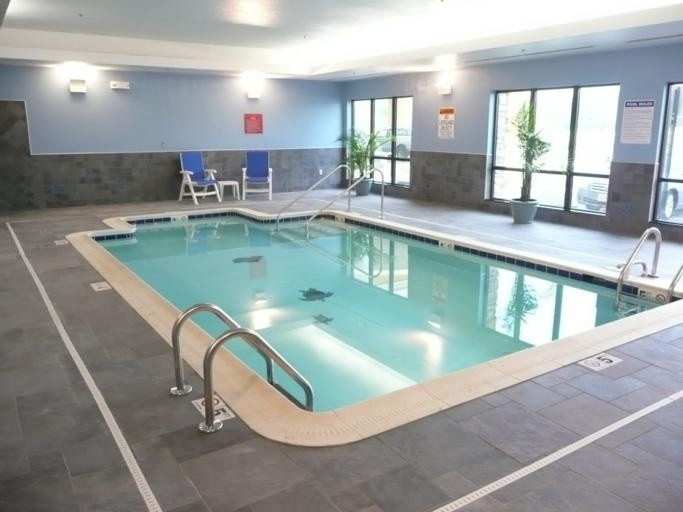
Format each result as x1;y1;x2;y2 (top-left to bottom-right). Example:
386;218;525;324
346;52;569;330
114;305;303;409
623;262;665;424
217;180;240;201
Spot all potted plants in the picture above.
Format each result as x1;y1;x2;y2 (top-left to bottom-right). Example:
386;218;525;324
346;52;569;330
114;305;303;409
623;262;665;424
333;127;402;195
503;100;552;223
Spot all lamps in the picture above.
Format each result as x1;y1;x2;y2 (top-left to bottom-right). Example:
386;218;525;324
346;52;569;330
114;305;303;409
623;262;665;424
69;78;86;93
439;83;453;95
247;88;260;99
253;294;267;306
428;313;442;329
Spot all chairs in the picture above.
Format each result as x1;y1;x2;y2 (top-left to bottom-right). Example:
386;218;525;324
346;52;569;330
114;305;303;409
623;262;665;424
178;151;221;206
241;150;273;200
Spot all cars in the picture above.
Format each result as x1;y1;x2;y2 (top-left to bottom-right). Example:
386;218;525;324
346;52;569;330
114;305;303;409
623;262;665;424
374;128;411;159
576;151;682;219
